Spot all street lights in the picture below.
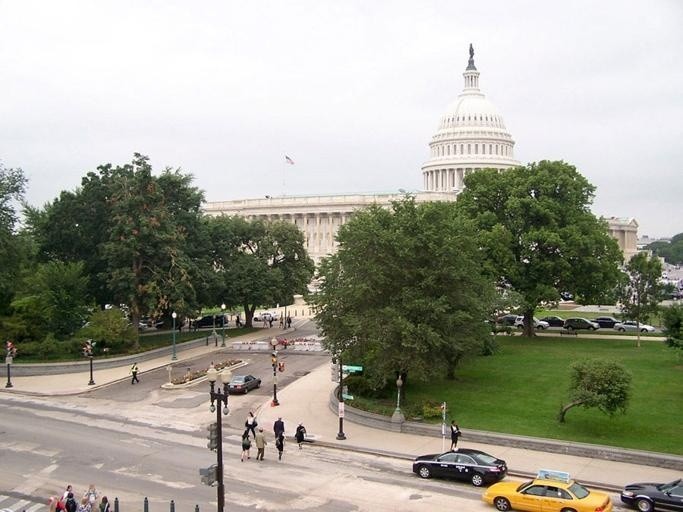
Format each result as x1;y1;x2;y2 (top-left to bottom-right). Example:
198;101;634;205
206;363;231;512
269;336;279;406
394;375;403;414
219;302;226;346
212;314;217;333
171;309;177;360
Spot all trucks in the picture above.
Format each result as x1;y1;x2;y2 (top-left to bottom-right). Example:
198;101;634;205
659;278;682;289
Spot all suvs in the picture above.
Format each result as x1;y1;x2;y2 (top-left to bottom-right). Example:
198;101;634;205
191;313;228;328
660;287;683;301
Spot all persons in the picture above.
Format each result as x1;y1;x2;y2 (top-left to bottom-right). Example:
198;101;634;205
262;316;268;329
49;485;112;512
269;314;273;328
244;412;258;441
450;419;462;450
129;361;140;384
286;316;291;328
255;427;268;461
273;417;286;440
295;423;307;450
278;315;283;329
275;435;286;461
240;430;253;463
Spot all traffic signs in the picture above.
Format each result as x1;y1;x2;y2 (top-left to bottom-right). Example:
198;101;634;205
341;364;362;371
342;394;354;401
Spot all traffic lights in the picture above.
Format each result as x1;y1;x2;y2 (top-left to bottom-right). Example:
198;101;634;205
198;464;216;487
12;348;17;357
80;338;96;359
270;349;277;368
6;342;12;355
329;363;339;382
206;422;216;451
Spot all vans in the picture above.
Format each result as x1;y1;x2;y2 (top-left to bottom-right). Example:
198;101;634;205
661;271;668;279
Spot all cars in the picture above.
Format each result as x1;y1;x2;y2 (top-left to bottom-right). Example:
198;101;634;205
412;448;506;485
620;478;683;512
481;469;612;511
489;312;656;337
154;320;185;329
252;311;278;321
126;322;147;332
560;292;572;300
227;373;261;394
